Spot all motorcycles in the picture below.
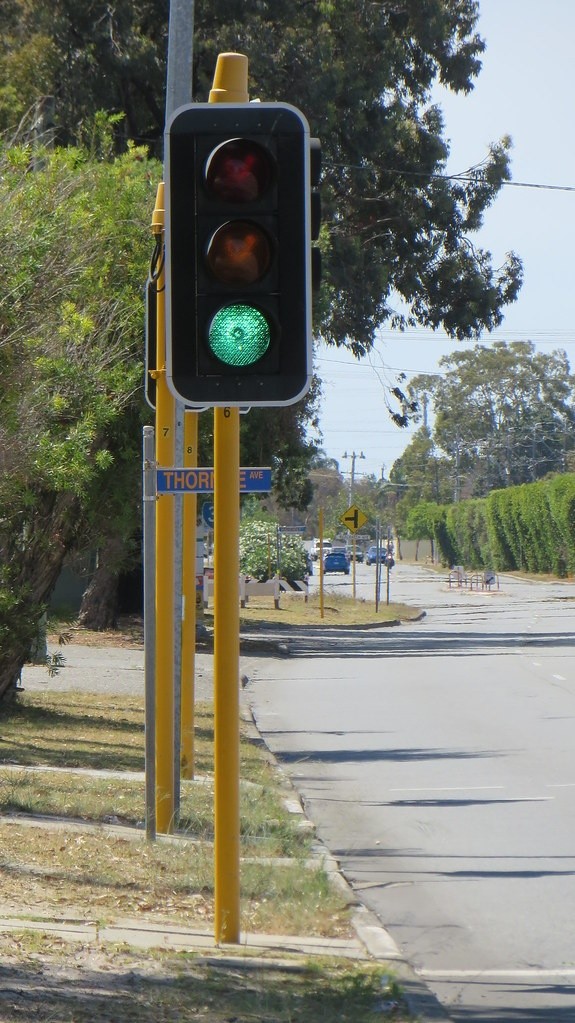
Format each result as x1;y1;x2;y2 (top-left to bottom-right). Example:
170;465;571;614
383;554;395;569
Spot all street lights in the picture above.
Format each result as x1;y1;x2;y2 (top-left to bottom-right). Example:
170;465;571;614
342;451;366;548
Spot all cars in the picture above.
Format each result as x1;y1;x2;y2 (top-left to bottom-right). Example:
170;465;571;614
331;547;346;554
365;547;388;565
323;553;349;574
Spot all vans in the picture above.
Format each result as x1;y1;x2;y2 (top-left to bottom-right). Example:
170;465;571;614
345;545;364;562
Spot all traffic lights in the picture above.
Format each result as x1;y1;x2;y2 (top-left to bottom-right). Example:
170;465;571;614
147;96;321;413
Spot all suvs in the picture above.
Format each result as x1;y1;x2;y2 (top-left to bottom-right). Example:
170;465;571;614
310;541;332;560
